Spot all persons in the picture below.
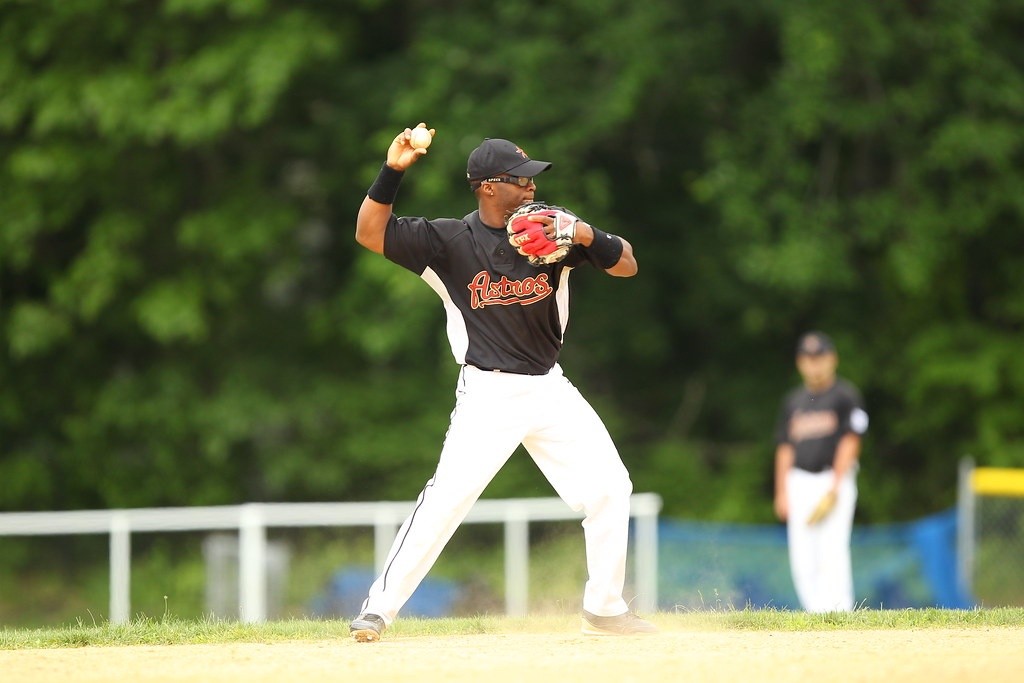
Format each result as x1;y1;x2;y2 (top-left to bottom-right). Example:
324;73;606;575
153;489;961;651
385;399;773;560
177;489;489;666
350;124;659;643
774;332;868;612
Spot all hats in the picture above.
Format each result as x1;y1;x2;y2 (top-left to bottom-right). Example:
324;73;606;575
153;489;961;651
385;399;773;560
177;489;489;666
797;330;834;355
467;138;552;178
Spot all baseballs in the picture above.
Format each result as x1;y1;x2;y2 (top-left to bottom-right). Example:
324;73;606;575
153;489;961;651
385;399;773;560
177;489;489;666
409;127;432;149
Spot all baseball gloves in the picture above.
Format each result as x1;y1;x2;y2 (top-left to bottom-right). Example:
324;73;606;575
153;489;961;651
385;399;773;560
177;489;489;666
507;202;582;265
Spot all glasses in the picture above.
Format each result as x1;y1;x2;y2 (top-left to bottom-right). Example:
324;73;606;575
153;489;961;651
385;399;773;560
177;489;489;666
488;176;534;187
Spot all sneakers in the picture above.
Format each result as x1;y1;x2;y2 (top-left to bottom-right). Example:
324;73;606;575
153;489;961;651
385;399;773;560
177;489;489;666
349;613;385;642
581;608;658;636
